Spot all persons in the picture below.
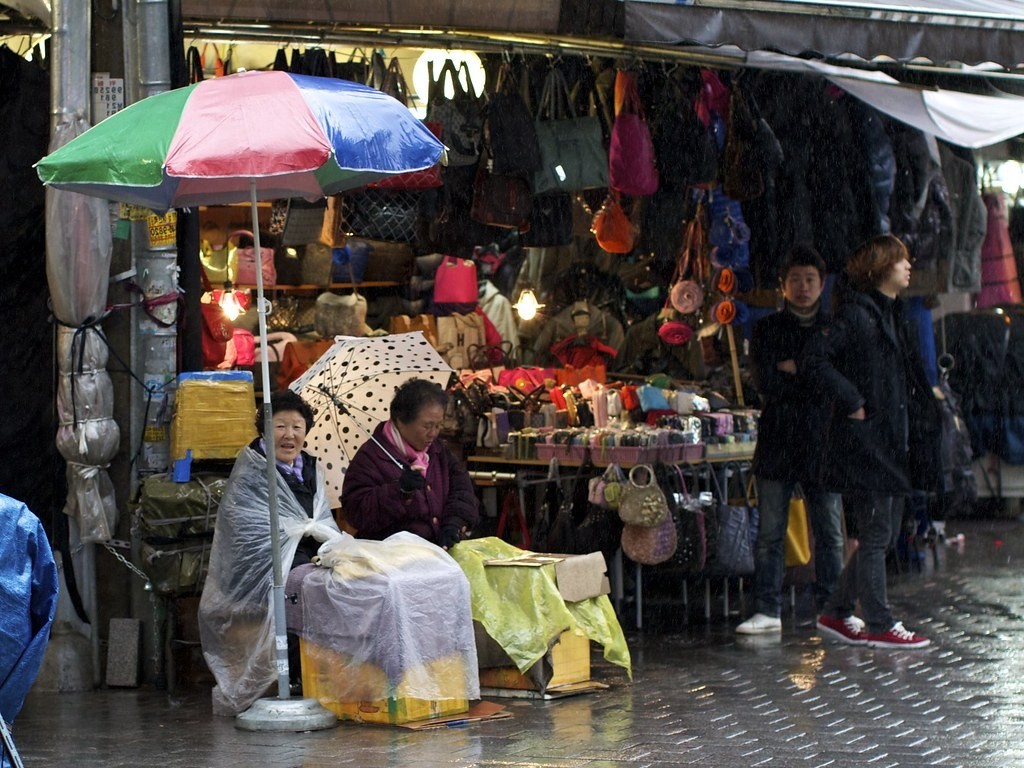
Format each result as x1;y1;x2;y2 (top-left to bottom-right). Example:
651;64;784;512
214;389;342;697
807;236;933;648
341;377;478;551
734;256;843;634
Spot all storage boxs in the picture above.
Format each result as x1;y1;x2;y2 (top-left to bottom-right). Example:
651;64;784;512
536;438;758;467
300;640;470;723
479;623;590;693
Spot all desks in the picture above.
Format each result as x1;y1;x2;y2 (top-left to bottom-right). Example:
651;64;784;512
469;455;754;644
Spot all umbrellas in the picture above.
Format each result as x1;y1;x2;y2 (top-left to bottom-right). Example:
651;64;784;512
286;331;456;510
33;66;452;699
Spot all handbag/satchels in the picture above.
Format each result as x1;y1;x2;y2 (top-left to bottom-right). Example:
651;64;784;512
181;38;811;579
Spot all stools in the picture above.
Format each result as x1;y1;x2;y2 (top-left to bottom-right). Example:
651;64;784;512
172;370;259;482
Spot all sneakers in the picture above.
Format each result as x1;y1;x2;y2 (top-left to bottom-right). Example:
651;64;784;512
868;621;931;648
847;614;865;629
816;613;869;645
735;613;782;635
